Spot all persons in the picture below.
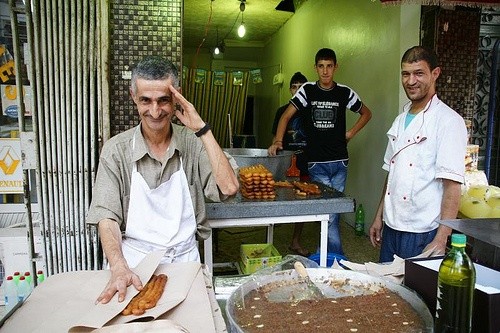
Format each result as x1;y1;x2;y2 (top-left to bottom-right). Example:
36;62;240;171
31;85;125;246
86;55;240;306
370;46;467;263
268;48;372;255
272;72;311;258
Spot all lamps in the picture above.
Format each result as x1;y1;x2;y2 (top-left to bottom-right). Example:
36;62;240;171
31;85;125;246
215;0;247;54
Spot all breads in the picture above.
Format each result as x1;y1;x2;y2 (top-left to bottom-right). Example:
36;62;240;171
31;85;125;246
292;180;321;194
121;274;167;314
238;164;276;199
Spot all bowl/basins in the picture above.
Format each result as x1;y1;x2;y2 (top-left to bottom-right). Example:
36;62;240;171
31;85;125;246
224;147;295;183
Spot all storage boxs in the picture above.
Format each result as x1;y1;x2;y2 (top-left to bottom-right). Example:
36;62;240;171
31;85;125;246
239;244;282;275
404;256;500;333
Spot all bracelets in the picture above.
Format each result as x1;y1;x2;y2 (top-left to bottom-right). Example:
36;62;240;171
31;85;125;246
195;122;210;137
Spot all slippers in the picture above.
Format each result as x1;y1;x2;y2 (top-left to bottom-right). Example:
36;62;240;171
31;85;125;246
288;246;311;257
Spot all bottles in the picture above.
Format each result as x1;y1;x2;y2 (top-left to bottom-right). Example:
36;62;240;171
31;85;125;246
433;233;477;333
354;204;365;236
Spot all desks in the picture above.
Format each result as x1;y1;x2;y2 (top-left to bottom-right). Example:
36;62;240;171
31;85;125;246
435;217;500;272
203;176;355;280
0;263;227;333
